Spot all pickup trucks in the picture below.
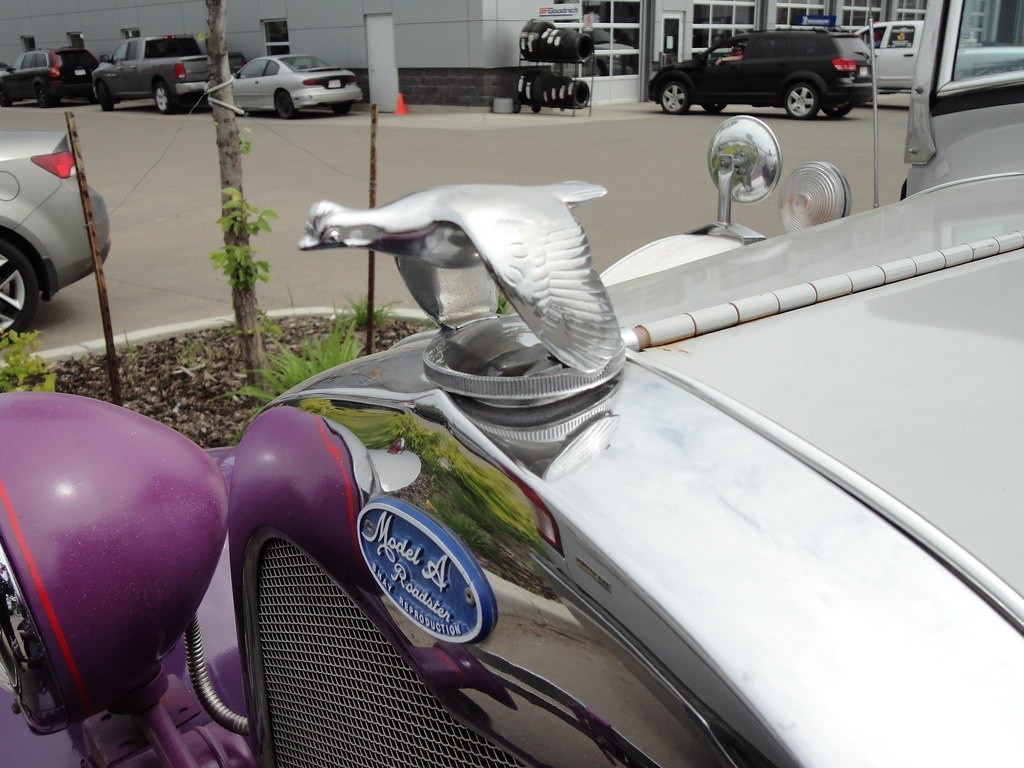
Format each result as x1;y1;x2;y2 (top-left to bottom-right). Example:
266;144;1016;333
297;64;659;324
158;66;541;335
92;35;245;115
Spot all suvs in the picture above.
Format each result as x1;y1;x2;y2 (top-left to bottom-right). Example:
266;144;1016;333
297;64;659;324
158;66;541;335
650;29;874;119
0;46;98;108
568;20;634;76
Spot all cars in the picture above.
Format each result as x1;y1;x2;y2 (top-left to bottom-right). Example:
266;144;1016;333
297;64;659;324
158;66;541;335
0;0;1024;768
0;131;112;345
208;54;361;117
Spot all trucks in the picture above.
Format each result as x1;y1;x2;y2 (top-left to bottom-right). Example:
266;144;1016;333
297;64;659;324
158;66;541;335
854;21;1023;102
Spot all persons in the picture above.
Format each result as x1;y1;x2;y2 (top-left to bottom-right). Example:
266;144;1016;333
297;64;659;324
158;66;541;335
715;44;745;66
867;31;883;49
